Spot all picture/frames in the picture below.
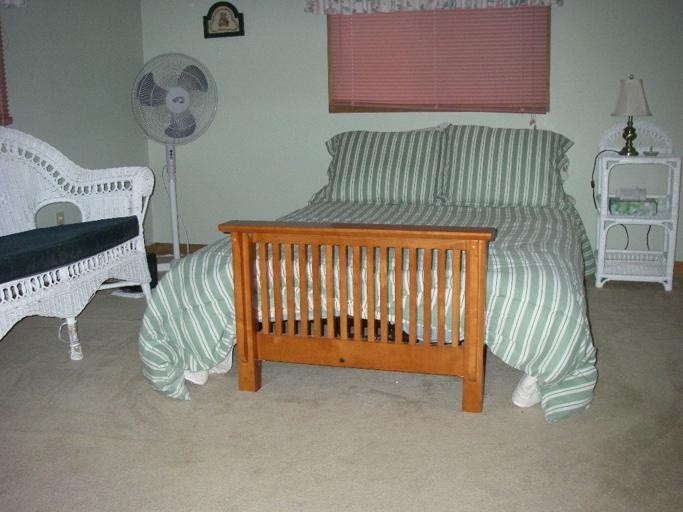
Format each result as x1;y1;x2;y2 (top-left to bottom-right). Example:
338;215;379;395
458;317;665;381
203;2;244;38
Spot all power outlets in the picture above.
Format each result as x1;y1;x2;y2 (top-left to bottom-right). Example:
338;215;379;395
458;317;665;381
57;212;64;226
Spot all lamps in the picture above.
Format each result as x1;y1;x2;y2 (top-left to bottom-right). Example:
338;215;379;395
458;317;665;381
611;75;651;156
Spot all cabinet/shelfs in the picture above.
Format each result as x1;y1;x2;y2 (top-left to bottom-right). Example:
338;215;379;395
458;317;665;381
595;123;681;291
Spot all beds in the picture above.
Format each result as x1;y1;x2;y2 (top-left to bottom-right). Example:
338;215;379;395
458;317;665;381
218;221;495;412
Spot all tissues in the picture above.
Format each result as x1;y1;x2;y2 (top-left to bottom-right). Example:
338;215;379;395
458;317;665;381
609;187;658;216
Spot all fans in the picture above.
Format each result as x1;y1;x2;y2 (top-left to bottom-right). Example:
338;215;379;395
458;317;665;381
131;54;219;272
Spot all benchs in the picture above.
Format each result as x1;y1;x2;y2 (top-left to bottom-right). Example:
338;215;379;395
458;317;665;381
0;127;156;360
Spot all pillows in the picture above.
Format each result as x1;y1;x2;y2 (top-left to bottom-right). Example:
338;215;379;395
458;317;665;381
433;124;575;209
308;131;447;206
